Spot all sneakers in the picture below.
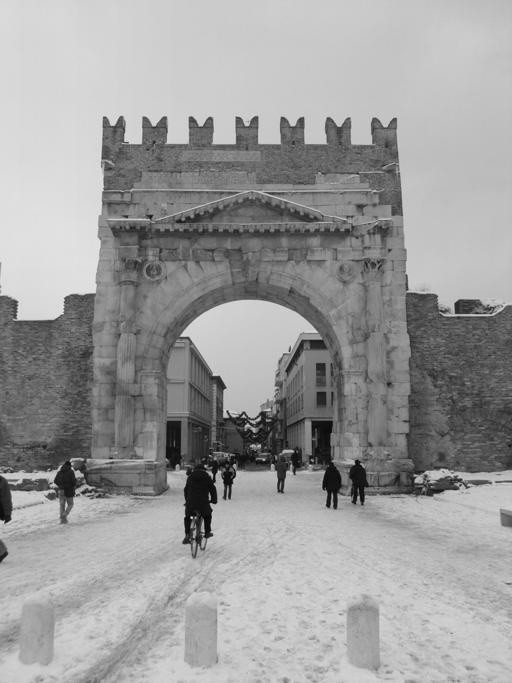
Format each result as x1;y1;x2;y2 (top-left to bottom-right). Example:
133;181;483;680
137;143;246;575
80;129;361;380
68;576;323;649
204;533;214;539
182;536;191;544
0;551;9;563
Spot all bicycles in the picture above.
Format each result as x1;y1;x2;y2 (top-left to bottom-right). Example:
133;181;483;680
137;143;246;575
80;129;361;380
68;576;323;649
183;499;212;560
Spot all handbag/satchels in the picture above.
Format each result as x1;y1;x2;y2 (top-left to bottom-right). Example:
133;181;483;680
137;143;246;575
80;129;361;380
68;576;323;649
63;482;76;498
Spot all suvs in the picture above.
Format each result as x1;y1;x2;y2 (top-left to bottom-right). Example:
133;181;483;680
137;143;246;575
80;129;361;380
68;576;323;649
280;448;301;462
255;451;275;464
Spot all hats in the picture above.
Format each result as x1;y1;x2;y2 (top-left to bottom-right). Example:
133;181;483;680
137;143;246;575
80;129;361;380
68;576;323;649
194;464;207;471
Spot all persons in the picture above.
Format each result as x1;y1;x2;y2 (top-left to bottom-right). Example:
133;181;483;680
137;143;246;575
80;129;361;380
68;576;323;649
54;461;77;523
186;456;236;500
275;457;287;493
291;451;298;475
0;475;12;563
182;463;217;544
322;461;341;510
350;460;368;506
